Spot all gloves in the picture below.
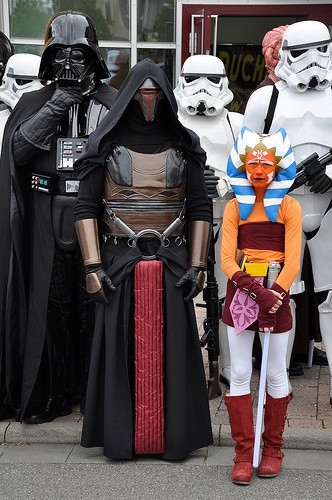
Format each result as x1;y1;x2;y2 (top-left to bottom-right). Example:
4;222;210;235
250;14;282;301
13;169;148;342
84;263;116;306
308;169;332;194
19;82;83;150
203;170;220;194
175;266;206;303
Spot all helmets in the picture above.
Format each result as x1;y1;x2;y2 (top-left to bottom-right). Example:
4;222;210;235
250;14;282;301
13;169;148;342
275;21;332;92
172;54;234;117
37;10;112;86
0;54;50;111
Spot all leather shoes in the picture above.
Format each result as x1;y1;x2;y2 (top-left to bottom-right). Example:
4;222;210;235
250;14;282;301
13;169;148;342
24;395;72;424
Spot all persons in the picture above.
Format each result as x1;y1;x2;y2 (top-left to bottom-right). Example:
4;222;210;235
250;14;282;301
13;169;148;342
0;10;119;424
220;128;303;485
174;54;244;399
242;21;332;404
74;58;213;463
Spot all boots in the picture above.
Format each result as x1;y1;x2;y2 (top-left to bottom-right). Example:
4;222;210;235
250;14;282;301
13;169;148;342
257;391;293;477
224;392;253;485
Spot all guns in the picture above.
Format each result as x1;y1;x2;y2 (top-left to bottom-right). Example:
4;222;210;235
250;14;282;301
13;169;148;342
286;149;331;196
